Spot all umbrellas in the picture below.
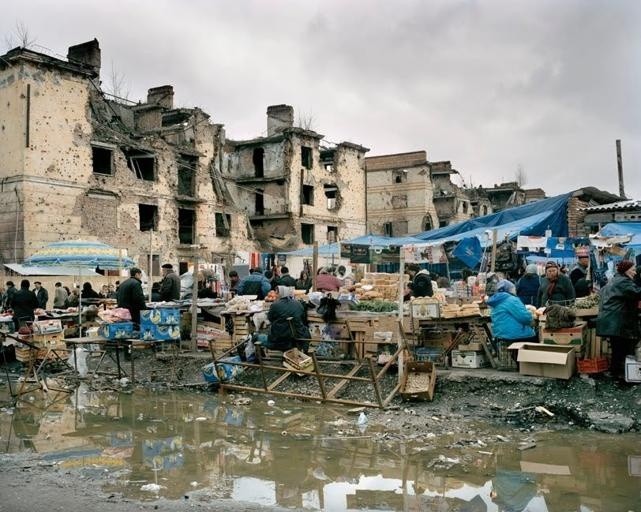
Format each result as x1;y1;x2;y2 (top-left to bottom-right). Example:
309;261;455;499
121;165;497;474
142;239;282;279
22;236;137;339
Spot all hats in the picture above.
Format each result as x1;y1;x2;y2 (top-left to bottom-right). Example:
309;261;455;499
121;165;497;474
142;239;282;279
618;262;634;274
162;264;172;268
496;279;515;292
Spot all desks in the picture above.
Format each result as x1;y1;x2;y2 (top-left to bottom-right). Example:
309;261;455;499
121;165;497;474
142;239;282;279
60;336;164;384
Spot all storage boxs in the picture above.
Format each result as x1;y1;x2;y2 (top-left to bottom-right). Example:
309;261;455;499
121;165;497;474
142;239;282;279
538;318;641;384
200;355;243;383
506;342;577;380
397;360;436;402
281;348;314;376
190;308;451;368
451;349;491;369
13;308;180;363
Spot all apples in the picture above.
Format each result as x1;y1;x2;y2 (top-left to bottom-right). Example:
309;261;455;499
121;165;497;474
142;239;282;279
525;305;543;319
265;290;277;300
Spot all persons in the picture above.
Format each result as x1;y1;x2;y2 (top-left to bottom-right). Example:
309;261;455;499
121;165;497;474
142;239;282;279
403;264;435;302
0;281;120;331
255;284;312;356
229;265;354;294
484;251;641;378
116;263;181;328
